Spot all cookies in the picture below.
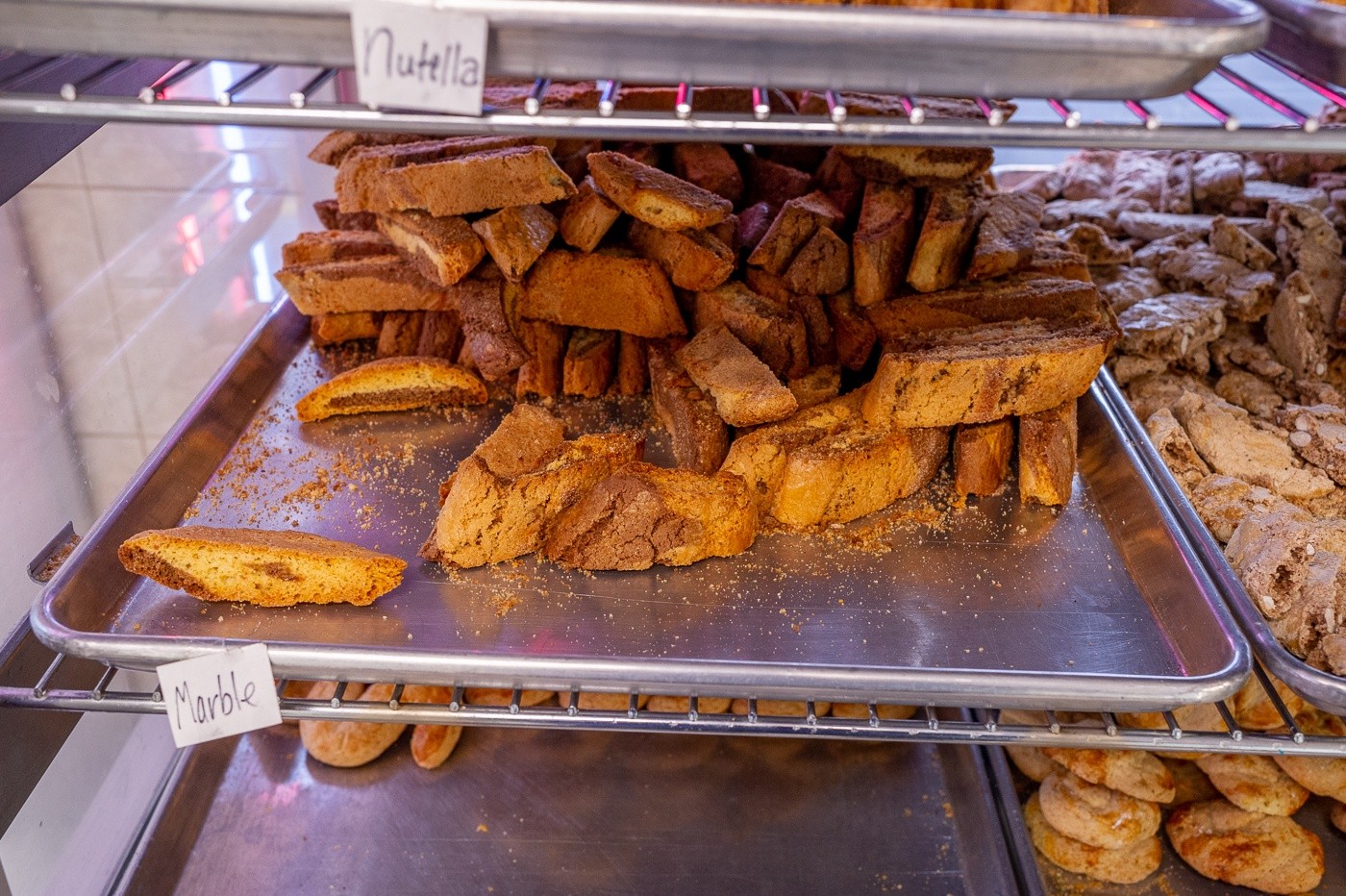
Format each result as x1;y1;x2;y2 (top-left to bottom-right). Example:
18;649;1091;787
997;670;1345;896
1008;106;1346;686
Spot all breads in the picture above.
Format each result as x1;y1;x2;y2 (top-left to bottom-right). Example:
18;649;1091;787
274;677;910;768
114;78;1126;606
